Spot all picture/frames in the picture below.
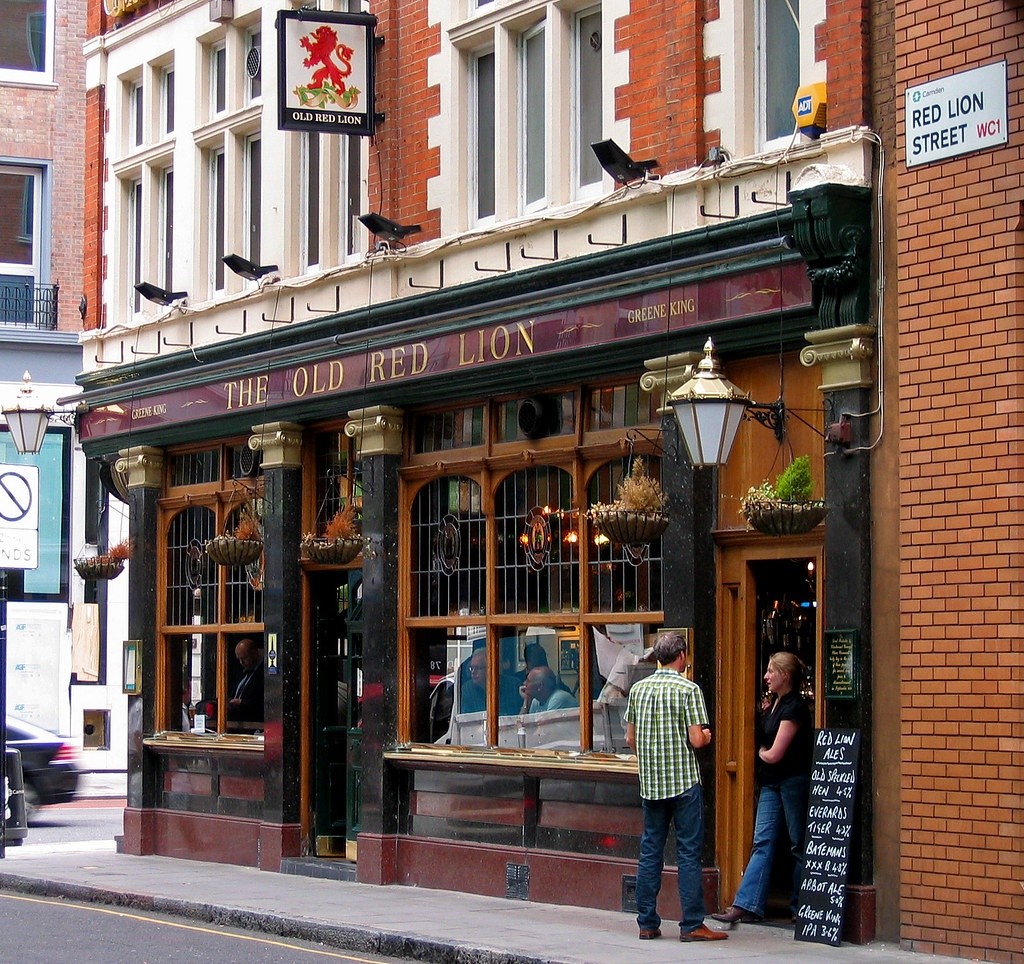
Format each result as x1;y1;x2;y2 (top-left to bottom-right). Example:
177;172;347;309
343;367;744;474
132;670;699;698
557;636;580;675
518;629;538;662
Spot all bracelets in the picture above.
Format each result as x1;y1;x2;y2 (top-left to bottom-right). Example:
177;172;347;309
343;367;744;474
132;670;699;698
524;705;530;710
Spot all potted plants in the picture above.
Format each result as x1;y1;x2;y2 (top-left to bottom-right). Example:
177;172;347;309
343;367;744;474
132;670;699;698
204;509;264;567
580;455;671;546
72;538;136;580
741;452;831;536
298;504;366;566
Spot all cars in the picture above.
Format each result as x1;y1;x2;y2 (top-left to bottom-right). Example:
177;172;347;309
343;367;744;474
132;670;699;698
4;712;81;824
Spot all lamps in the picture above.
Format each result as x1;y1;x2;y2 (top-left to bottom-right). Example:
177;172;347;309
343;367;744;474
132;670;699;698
132;138;659;305
0;370;89;455
666;336;785;470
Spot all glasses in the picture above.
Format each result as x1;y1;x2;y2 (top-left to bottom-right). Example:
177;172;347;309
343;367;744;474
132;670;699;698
468;665;487;672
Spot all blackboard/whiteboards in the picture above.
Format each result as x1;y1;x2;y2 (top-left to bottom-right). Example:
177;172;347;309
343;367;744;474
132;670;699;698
791;726;865;948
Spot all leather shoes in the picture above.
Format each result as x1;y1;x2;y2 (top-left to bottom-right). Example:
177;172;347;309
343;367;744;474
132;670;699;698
679;924;729;942
638;928;662;940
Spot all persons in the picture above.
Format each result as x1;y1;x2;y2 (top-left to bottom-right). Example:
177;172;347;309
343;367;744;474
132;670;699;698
620;630;728;942
708;652;815;924
127;693;144;766
457;639;581;715
226;639;265;724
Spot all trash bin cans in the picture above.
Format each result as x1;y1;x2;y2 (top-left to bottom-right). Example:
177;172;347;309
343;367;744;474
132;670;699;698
5;746;29;847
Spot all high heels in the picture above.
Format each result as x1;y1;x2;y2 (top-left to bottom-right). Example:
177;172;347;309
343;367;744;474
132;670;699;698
712;906;759;923
